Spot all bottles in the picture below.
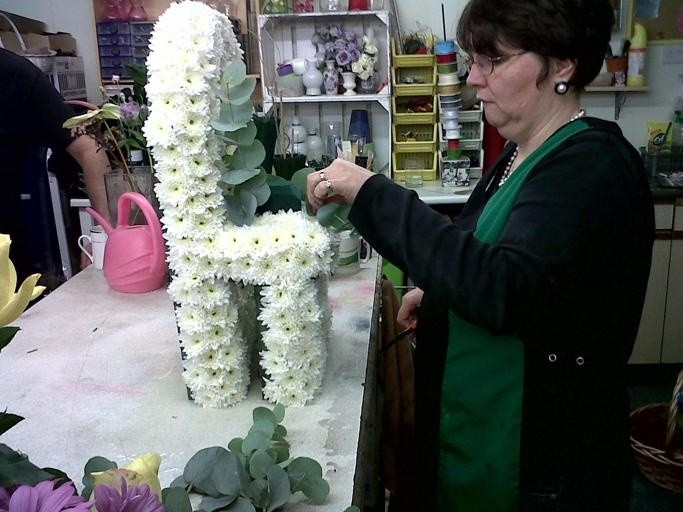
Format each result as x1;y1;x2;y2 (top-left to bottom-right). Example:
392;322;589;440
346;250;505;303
286;115;306;157
327;125;342;159
305;128;327;165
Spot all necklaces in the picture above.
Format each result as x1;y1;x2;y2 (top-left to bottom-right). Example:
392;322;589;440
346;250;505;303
496;109;585;186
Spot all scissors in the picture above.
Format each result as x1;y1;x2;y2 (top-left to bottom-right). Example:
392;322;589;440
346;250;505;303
377;317;421;354
355;137;367;157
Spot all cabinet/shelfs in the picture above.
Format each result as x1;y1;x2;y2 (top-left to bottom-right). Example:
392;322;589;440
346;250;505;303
440;99;484;179
255;7;391;196
393;32;438;182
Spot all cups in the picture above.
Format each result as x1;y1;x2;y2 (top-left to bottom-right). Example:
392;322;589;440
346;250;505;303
403;158;425;188
334;229;371;276
78;225;110;270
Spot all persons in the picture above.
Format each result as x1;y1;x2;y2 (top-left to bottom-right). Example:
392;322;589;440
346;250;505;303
0;46;114;312
306;2;658;512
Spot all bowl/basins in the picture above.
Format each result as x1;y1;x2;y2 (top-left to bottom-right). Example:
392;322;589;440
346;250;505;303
18;49;58;74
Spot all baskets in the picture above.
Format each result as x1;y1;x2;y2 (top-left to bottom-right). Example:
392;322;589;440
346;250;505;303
628;380;683;489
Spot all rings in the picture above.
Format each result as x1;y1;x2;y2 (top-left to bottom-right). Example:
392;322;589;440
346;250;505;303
319;171;325;182
325;180;333;193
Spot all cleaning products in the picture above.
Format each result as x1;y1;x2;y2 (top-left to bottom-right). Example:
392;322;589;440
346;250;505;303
626;23;649;87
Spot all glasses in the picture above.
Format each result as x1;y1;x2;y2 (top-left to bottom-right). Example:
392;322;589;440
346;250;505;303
464;51;527;76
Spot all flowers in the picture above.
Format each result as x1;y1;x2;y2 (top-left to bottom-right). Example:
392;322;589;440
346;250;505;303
63;0;337;415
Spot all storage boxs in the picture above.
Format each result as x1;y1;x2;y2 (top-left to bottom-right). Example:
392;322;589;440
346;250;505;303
0;10;77;56
